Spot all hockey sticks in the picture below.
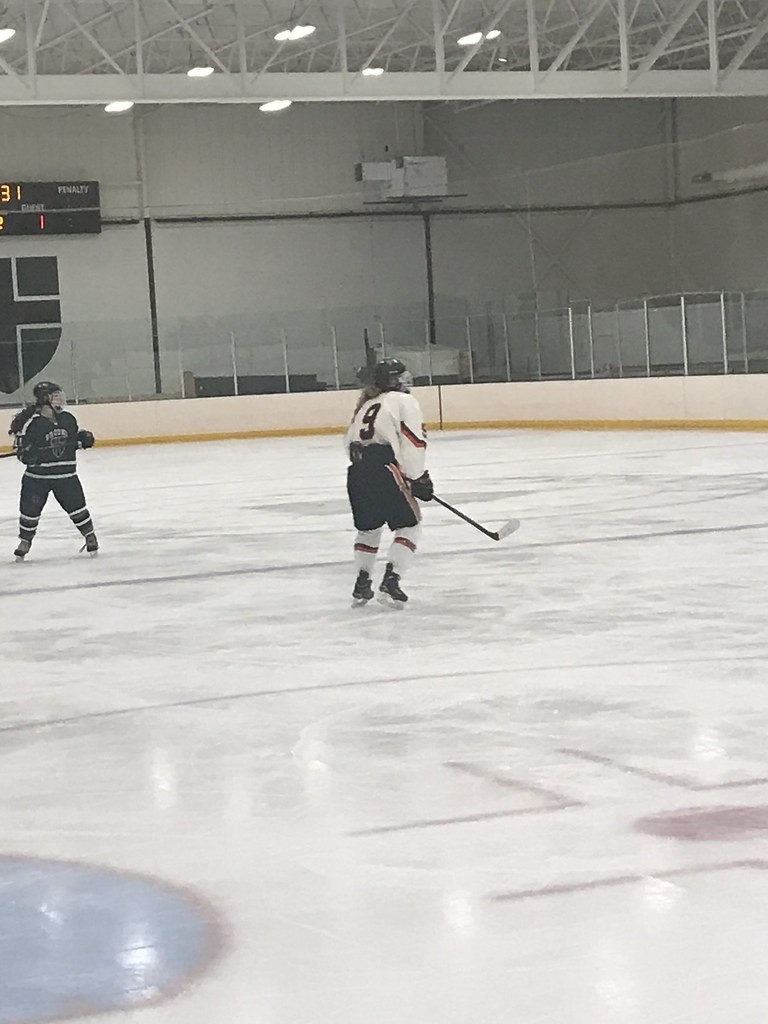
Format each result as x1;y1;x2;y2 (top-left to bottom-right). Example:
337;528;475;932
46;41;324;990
0;440;84;459
406;476;523;542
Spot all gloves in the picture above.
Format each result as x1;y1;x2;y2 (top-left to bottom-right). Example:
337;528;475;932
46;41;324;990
411;470;433;502
16;443;40;465
77;429;95;450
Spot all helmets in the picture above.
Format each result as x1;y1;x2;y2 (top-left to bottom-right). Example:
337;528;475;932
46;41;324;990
32;381;67;414
375;357;414;392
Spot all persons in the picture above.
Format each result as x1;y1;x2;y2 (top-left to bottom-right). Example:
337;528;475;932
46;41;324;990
344;357;435;603
7;381;99;557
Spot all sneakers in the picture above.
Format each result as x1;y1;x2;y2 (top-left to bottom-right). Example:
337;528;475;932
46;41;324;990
13;541;32;563
378;563;408;610
84;532;100;557
351;570;374;608
